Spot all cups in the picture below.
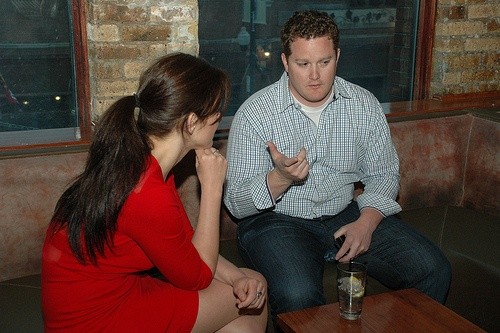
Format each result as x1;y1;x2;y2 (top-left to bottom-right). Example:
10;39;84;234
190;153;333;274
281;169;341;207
337;262;368;321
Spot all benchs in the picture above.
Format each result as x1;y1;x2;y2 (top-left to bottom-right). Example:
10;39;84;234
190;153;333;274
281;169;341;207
0;206;500;333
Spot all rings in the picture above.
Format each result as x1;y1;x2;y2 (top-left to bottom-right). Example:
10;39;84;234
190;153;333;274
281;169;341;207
212;150;220;156
257;291;262;297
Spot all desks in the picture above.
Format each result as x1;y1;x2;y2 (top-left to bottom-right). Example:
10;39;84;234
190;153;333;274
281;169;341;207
276;288;489;333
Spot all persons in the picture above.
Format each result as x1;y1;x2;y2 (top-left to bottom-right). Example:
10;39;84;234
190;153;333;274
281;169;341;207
223;10;452;333
42;53;268;333
